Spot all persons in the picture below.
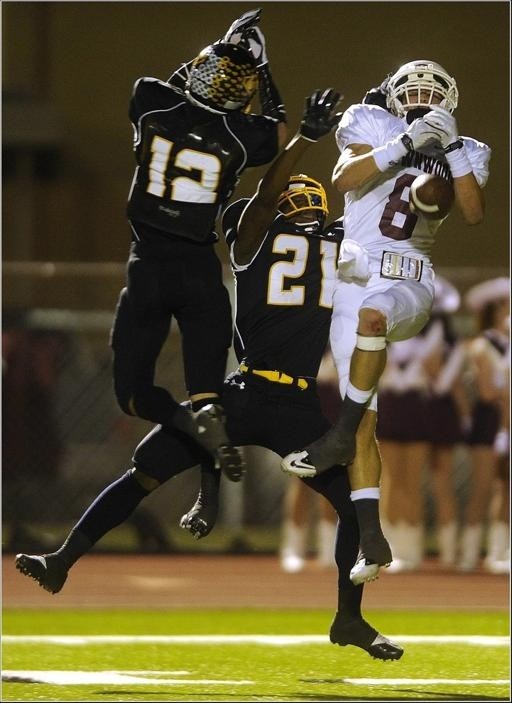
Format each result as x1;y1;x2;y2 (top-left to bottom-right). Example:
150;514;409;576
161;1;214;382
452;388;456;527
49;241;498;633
103;6;292;539
8;81;410;666
1;272;511;579
275;54;493;588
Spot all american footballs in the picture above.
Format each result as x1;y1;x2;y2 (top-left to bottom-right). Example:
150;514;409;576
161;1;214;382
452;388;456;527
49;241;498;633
409;173;454;221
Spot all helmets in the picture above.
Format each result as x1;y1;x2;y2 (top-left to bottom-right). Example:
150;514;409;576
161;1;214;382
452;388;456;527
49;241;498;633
385;59;459;120
275;173;330;235
184;43;259;113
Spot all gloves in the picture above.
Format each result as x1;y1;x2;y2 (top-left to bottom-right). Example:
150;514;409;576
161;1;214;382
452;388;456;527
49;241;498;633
371;116;442;174
423;103;473;179
213;8;263;46
238;25;288;124
299;86;344;144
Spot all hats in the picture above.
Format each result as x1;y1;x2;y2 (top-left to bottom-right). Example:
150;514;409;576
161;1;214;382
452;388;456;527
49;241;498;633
427;276;510;316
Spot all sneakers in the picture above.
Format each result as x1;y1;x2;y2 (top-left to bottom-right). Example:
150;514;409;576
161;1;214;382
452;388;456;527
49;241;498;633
330;611;403;662
280;427;356;478
179;500;217;540
194;403;245;483
349;538;393;587
15;553;68;595
